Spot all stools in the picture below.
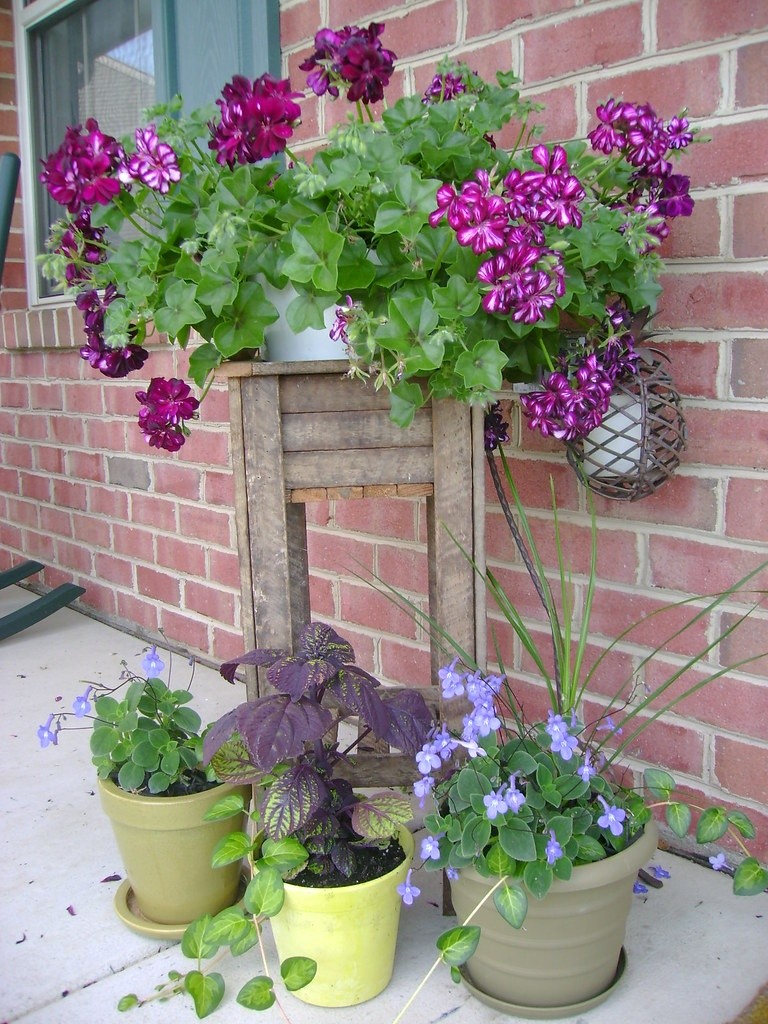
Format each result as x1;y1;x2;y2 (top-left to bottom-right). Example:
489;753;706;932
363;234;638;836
210;360;488;822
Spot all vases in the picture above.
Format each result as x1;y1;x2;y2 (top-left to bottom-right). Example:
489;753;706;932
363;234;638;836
253;257;388;361
433;822;658;1017
91;768;249;940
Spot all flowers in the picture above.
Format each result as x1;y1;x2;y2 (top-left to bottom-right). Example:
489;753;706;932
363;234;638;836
35;627;217;802
345;438;768;971
31;21;712;450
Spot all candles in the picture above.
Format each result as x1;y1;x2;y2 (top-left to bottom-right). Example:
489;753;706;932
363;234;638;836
582;392;649;477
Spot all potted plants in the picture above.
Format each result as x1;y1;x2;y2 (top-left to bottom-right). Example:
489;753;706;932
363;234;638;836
202;621;433;1009
